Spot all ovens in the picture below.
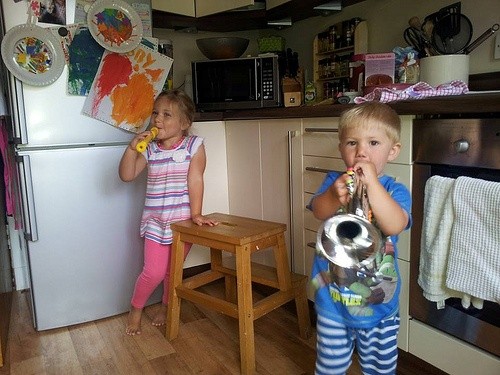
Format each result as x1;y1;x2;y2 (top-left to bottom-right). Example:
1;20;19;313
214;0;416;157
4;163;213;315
410;112;500;361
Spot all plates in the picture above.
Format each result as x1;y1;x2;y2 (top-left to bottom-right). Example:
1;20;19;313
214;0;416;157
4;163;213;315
1;24;67;86
87;0;143;53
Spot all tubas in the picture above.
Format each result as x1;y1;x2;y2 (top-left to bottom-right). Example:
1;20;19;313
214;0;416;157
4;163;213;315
315;165;383;270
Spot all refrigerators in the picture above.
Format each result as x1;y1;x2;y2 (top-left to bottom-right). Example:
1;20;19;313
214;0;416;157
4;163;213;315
0;0;167;333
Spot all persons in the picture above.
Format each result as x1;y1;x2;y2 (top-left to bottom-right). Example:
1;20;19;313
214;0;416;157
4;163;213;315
119;87;219;336
310;102;413;375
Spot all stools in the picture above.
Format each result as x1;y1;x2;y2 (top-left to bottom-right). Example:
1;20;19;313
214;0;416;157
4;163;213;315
166;212;312;375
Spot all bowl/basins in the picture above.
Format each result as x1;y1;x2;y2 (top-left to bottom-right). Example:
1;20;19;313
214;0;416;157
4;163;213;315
419;53;470;92
342;92;359;104
197;36;250;61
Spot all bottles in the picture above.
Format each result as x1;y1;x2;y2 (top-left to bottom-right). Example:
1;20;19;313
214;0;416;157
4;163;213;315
317;17;361;100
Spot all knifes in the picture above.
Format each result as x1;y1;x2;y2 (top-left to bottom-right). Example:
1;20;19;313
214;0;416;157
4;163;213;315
276;48;300;79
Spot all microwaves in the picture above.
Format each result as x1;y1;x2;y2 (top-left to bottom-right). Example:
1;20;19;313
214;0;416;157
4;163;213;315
190;53;287;114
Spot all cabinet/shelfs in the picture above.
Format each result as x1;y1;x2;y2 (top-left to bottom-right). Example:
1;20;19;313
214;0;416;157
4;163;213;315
314;19;368;102
302;115;413;352
224;118;305;279
184;121;226;268
152;0;355;33
